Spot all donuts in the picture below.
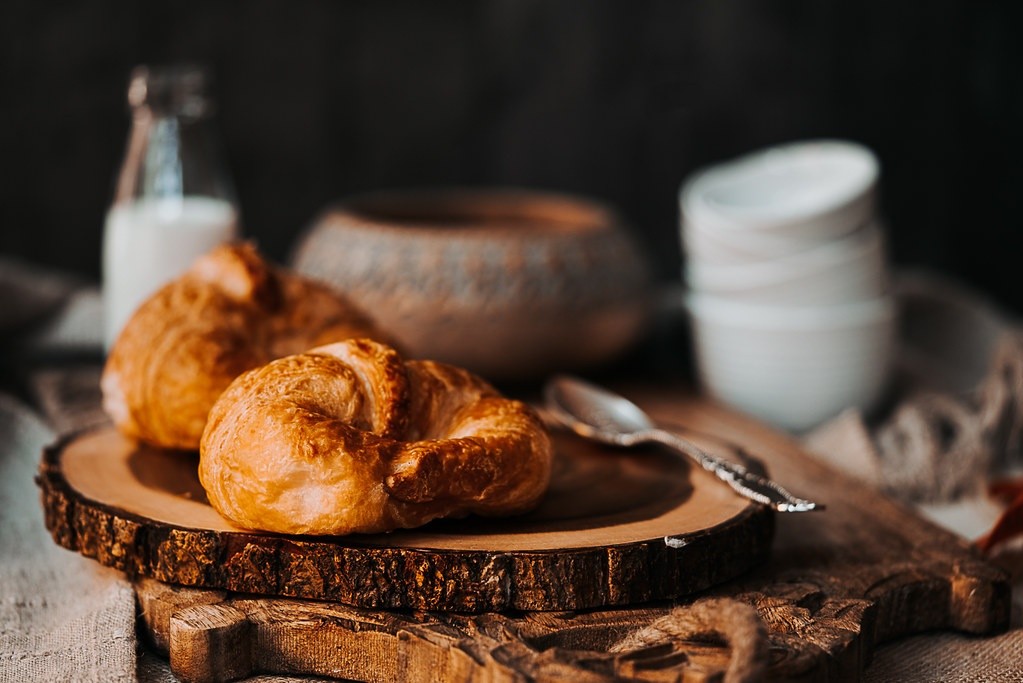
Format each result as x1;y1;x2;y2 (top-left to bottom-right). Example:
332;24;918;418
196;340;554;538
101;240;405;453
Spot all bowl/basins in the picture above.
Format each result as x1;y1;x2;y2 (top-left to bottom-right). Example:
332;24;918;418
679;140;904;434
299;190;649;374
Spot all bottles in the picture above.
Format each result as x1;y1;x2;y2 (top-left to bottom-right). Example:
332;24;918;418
95;69;234;348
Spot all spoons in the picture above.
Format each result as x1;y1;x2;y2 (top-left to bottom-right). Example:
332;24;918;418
548;376;825;516
